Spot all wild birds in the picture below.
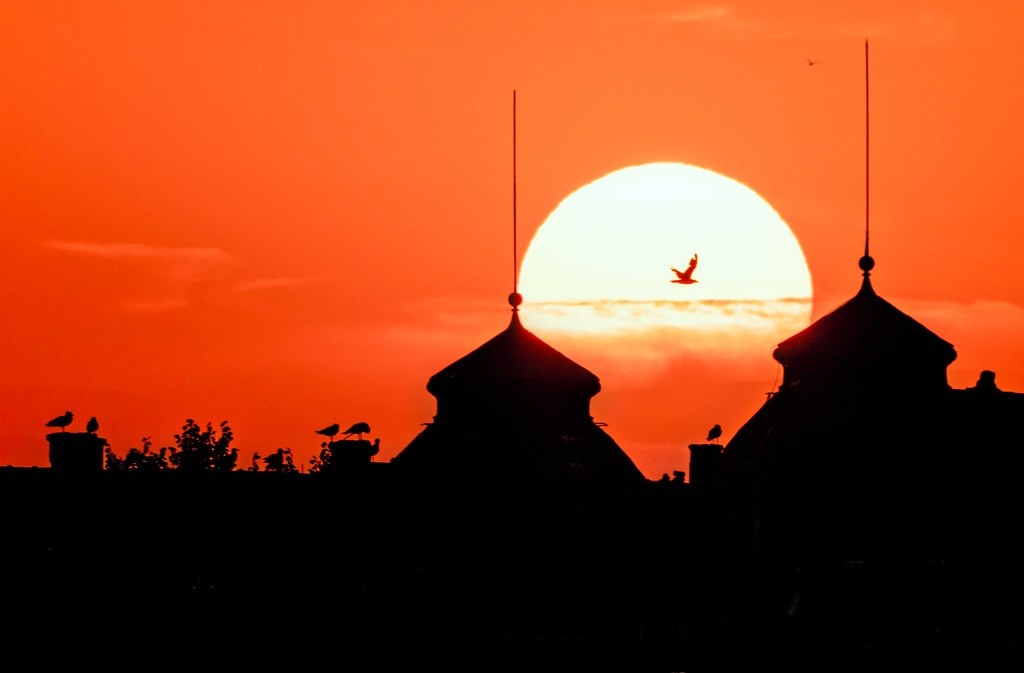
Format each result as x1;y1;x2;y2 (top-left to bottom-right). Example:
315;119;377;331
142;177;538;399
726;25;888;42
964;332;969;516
87;418;98;433
705;424;722;445
315;423;339;442
976;370;1001;394
329;439;380;463
659;471;684;484
48;412;74;432
265;449;284;468
341;421;370;436
671;253;698;285
